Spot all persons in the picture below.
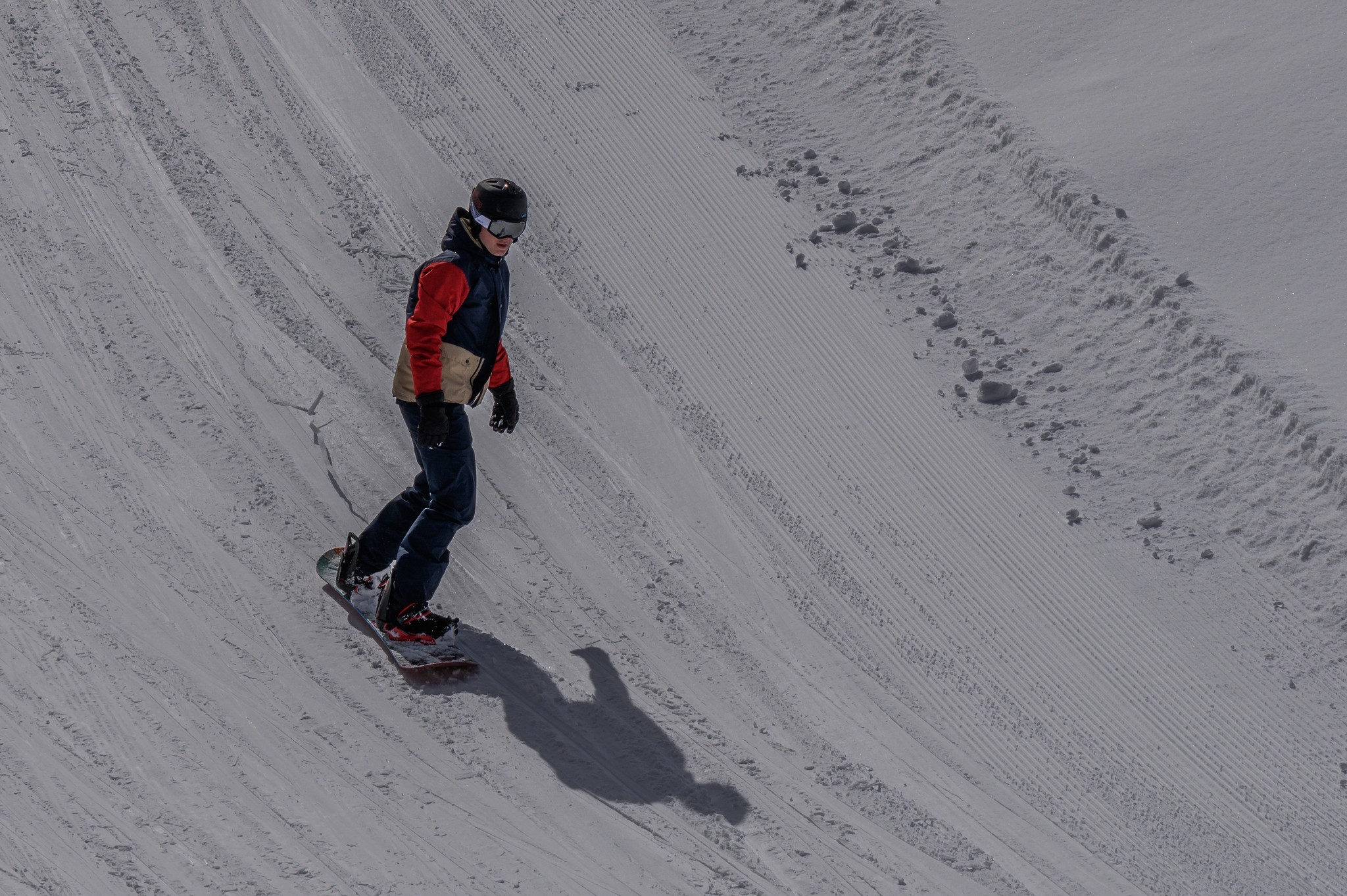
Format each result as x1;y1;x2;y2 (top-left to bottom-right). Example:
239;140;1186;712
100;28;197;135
336;177;528;644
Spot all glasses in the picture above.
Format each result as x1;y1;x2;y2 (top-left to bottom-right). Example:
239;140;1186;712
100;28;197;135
476;214;527;241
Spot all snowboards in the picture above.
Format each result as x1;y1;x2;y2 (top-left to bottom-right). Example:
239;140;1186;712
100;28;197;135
316;546;480;670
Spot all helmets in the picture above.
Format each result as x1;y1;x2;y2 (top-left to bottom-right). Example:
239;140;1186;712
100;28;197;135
469;178;528;234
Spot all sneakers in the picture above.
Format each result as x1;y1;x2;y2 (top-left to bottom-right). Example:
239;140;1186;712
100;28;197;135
376;603;454;641
338;559;391;593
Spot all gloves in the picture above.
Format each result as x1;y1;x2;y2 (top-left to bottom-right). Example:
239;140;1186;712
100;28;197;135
413;390;449;449
487;377;520;434
